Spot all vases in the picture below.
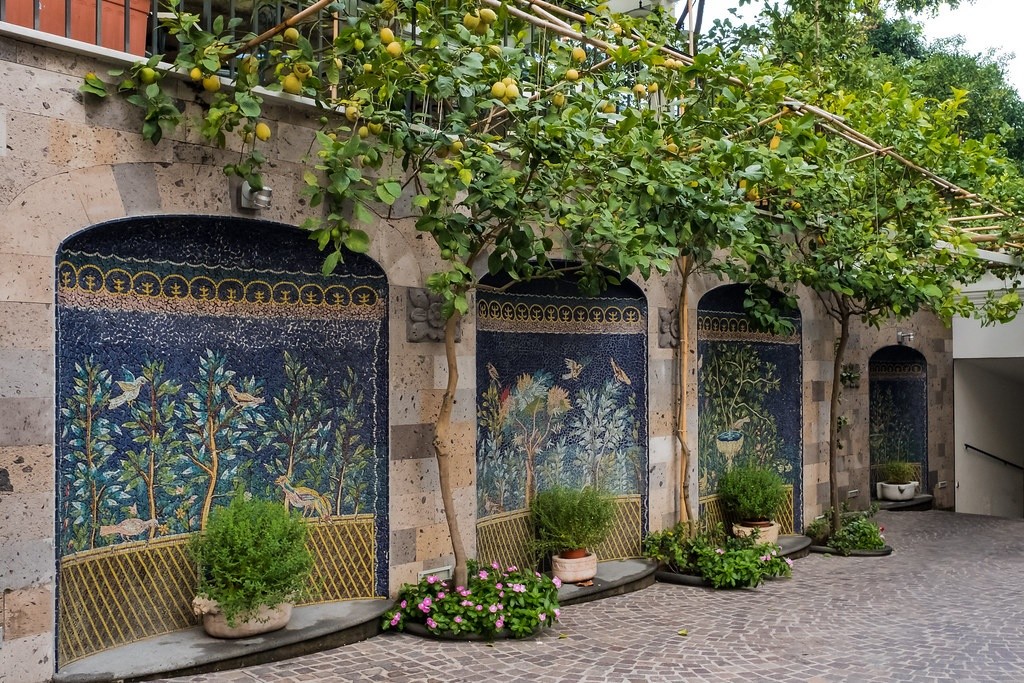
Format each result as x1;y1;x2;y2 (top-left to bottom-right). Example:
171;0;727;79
732;523;784;549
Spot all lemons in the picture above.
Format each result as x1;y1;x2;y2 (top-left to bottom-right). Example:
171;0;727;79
140;6;939;283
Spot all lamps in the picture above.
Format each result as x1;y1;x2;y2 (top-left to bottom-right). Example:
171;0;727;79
237;178;274;211
897;331;915;344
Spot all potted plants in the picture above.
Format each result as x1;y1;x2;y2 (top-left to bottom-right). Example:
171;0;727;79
877;458;920;502
522;480;622;574
716;454;789;527
183;482;339;637
551;552;597;584
